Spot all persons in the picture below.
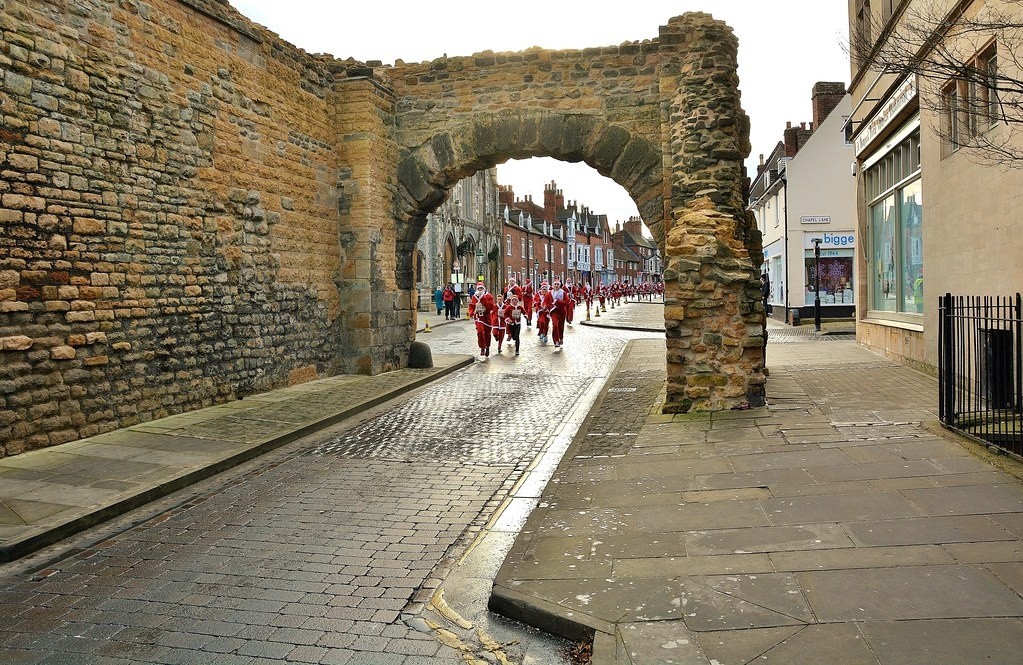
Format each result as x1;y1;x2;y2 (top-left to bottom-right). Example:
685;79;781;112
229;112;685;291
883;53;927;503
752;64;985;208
468;277;664;357
434;282;456;320
905;274;923;313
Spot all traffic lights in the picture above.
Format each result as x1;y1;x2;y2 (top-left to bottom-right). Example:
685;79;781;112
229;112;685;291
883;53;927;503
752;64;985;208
543;270;548;279
573;260;577;270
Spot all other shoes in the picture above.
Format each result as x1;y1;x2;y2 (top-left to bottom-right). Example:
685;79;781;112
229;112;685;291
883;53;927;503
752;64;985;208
555;343;560;347
515;351;519;355
498;349;501;352
542;337;548;342
450;317;455;320
446;317;449;320
485;348;489;356
481;348;484;355
539;334;545;339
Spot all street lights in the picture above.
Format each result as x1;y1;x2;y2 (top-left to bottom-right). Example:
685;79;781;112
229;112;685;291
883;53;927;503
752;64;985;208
533;258;540;294
811;237;823;333
476;247;485;282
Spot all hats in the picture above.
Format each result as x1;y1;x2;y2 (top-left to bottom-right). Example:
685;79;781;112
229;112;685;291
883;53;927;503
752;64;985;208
508;276;516;282
511;295;519;304
552;279;561;286
525;279;532;285
477;282;486;290
541;282;550;289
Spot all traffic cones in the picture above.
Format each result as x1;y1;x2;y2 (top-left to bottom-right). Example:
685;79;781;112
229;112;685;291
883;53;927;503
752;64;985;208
617;299;620;306
595;306;601;317
601;303;607;312
464;309;471;321
586;309;591;321
422;317;432;332
611;300;614;308
